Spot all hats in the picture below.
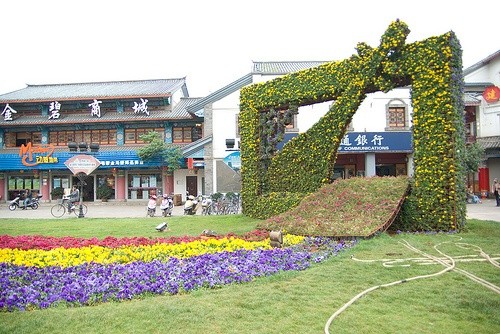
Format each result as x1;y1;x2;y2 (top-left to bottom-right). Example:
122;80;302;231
493;178;498;182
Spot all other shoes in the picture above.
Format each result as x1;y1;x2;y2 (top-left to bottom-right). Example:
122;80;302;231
71;205;75;209
22;208;25;210
25;208;27;210
66;212;71;214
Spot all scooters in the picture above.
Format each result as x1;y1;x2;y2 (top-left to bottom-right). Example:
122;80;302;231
159;191;176;217
183;191;200;216
9;193;43;211
146;190;160;217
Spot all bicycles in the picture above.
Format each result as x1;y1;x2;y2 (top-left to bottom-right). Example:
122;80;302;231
51;195;87;218
200;194;239;215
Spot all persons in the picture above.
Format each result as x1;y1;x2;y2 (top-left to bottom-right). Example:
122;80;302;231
22;189;32;210
67;185;80;214
466;185;478;203
492;178;500;206
